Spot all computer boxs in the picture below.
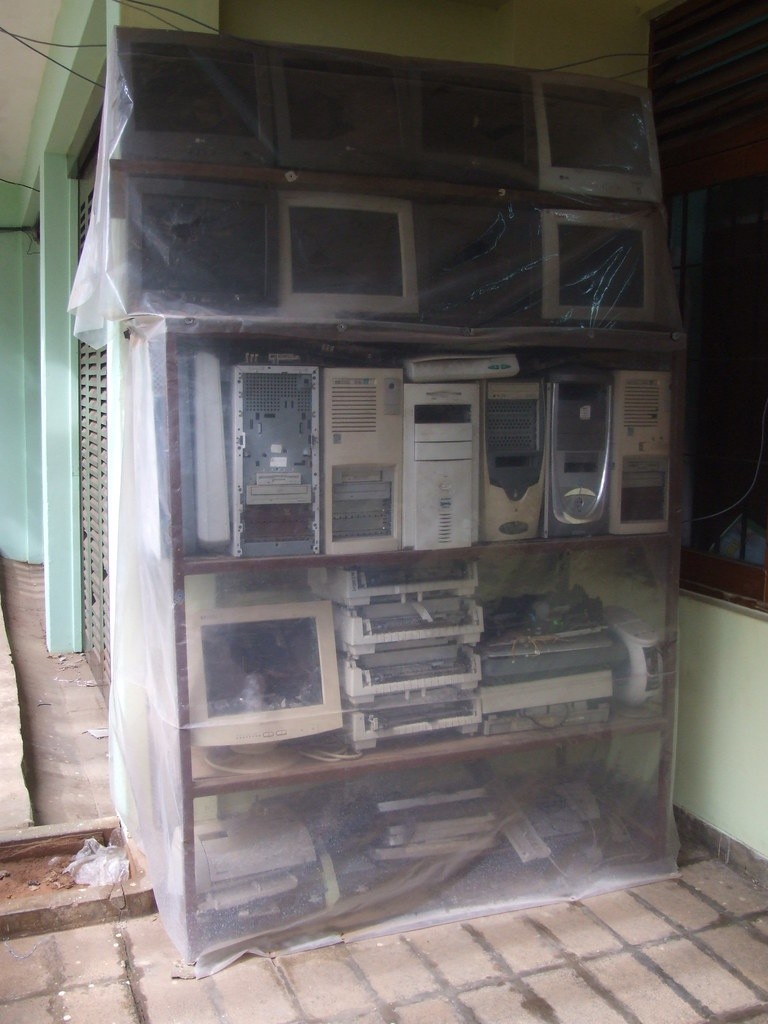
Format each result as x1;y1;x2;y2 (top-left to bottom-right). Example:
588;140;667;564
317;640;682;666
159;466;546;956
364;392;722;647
401;384;481;551
322;369;403;555
224;360;321;555
546;361;611;537
609;367;672;535
480;380;551;544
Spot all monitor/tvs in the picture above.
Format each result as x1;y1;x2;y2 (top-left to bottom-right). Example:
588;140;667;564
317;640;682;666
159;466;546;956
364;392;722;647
414;204;544;327
541;210;657;326
405;59;538;190
184;600;343;748
270;40;415;179
533;70;666;203
278;189;419;318
123;176;279;315
115;24;277;167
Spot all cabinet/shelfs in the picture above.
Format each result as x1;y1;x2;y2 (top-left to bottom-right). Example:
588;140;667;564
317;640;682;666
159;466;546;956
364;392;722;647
127;319;692;977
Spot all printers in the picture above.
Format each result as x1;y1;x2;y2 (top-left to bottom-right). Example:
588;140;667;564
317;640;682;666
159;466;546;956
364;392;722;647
173;808;318;892
